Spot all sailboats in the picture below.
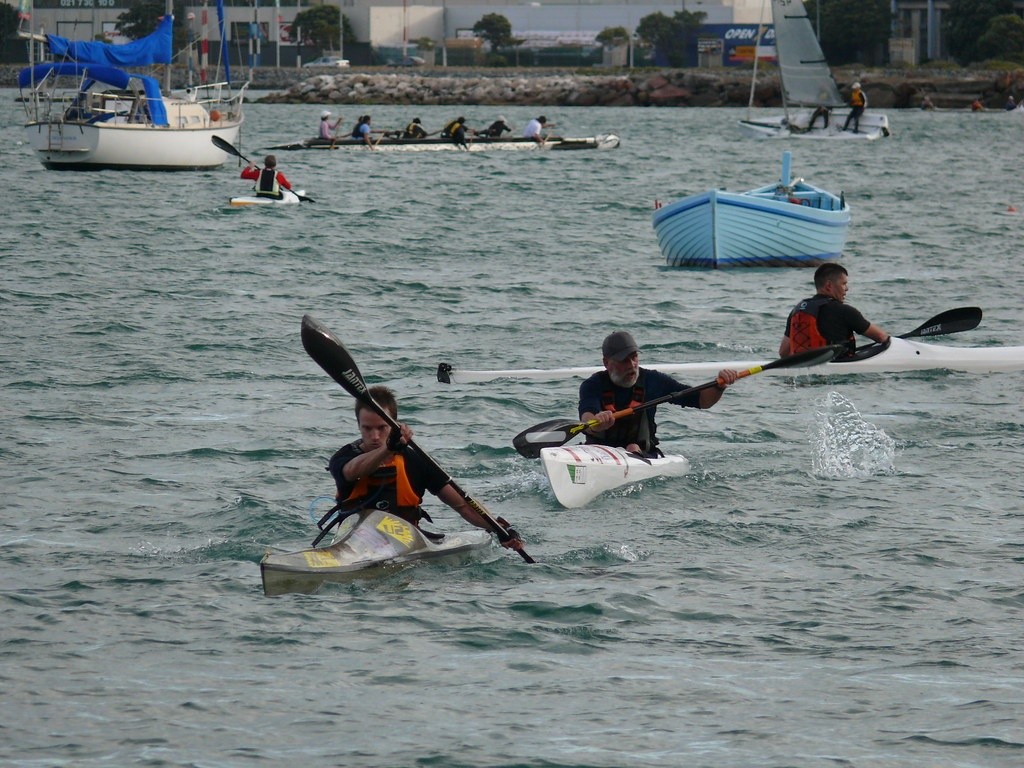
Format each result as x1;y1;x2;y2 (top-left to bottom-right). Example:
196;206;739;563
15;0;252;170
738;1;890;143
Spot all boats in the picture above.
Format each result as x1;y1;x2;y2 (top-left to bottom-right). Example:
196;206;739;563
540;443;691;508
229;189;306;209
260;509;508;599
653;150;850;268
261;134;620;151
437;336;1024;385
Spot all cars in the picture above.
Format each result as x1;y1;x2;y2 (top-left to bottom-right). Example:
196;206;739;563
303;56;350;68
387;56;425;67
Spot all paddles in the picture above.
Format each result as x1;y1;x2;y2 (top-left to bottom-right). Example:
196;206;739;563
468;130;476;151
329;117;344;152
512;342;842;459
300;313;536;563
210;134;317;204
855;305;984;352
371;132;385;150
539;126;554;150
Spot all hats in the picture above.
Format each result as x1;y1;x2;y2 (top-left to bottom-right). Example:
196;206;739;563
497;115;504;121
320;110;330;117
601;329;643;363
852;82;861;89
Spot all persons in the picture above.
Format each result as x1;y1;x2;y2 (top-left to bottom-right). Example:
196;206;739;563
523;115;556;142
241;155;291;200
779;263;887;357
579;331;737;459
319;111;341;139
921;95;933;110
489;115;515;137
972;98;984;111
843;82;867;132
441;115;477;146
403;117;428;138
1006;96;1016;111
332;388;523;551
352;115;373;149
808;86;832;128
